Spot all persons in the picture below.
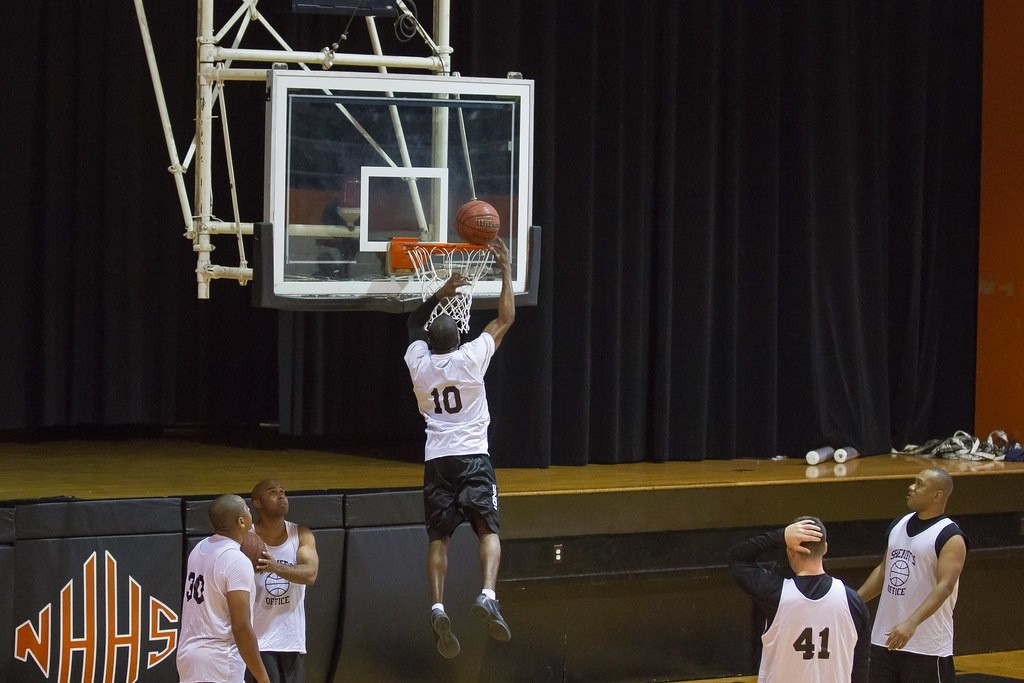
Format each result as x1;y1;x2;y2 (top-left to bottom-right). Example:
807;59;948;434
240;478;319;683
403;237;515;658
723;515;872;683
172;493;273;682
855;466;972;683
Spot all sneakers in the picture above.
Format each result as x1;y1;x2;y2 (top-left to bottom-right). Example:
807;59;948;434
430;608;460;659
472;594;511;641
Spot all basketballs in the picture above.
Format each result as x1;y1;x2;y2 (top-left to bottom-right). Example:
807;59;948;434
454;199;501;245
